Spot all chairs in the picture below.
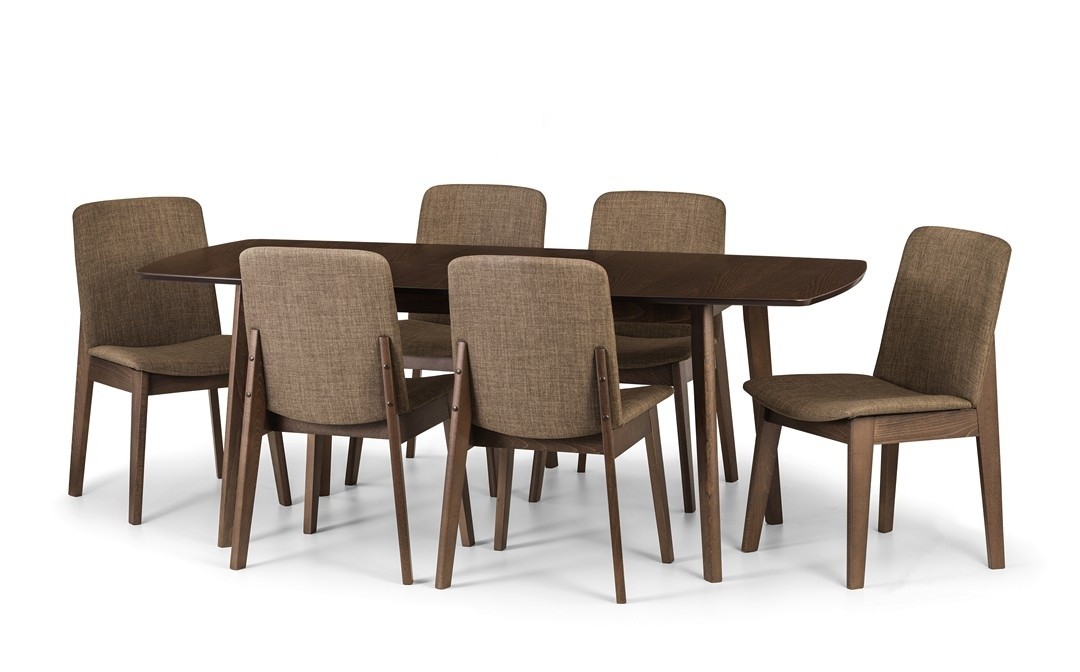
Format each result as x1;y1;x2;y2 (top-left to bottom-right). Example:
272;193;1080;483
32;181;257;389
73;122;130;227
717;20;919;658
68;197;233;525
435;255;675;604
230;246;475;584
345;184;546;497
740;227;1012;589
528;190;739;513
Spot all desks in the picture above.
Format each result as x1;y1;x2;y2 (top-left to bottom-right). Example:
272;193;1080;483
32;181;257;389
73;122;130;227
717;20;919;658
135;239;867;583
